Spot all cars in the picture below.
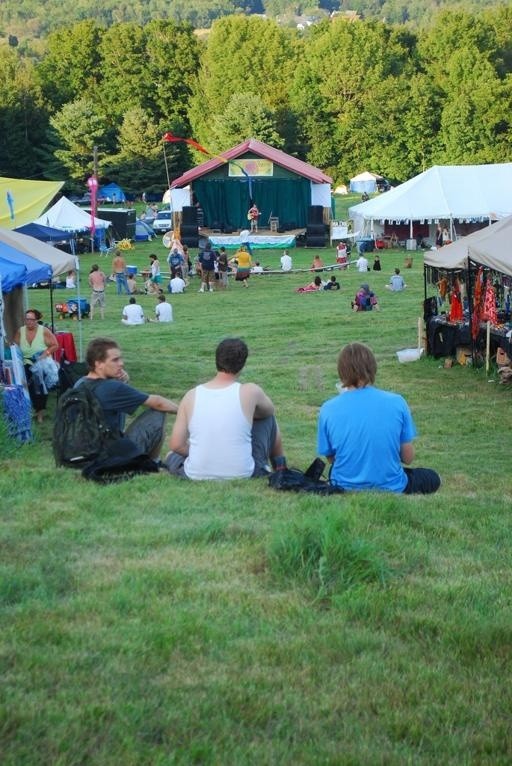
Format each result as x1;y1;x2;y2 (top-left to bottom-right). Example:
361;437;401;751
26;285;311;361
72;197;101;206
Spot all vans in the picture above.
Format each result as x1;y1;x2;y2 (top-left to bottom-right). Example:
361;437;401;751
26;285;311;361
152;210;172;234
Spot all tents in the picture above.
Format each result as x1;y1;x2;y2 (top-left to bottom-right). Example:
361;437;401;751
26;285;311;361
350;171;390;192
135;220;156;241
98;182;126;202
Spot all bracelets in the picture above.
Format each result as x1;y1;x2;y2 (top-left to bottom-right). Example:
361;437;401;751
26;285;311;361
46;351;51;356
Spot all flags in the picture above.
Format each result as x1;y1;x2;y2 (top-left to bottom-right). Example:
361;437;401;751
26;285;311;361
481;274;498;322
472;265;483;338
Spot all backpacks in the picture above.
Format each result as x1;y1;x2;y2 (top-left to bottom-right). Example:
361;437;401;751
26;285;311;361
52;379;109;468
268;455;341;492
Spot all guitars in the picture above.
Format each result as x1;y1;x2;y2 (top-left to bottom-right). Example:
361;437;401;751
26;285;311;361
247;212;262;220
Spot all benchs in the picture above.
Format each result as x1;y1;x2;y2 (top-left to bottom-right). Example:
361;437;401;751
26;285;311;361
139;260;360;275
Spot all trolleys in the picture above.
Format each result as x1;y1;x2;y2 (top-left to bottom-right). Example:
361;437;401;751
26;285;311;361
55;298;90;321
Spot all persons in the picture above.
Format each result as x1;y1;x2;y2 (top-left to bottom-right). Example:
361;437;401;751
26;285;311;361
101;190;158;219
385;267;408;292
12;309;59;423
318;342;441;495
357;253;369;271
436;223;442;245
336;240;351;270
298;275;321;290
73;338;179;470
321;275;340;290
351;283;380;311
248;203;259;233
310;254;324;271
374;255;381;270
362;191;369;201
443;224;449;242
61;242;264;326
280;250;293;272
164;337;288;481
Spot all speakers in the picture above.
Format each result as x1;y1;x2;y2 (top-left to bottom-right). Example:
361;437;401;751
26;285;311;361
306;234;325;247
306;224;325;236
181;237;199;248
182;206;197;225
180;224;198;237
308;205;323;225
